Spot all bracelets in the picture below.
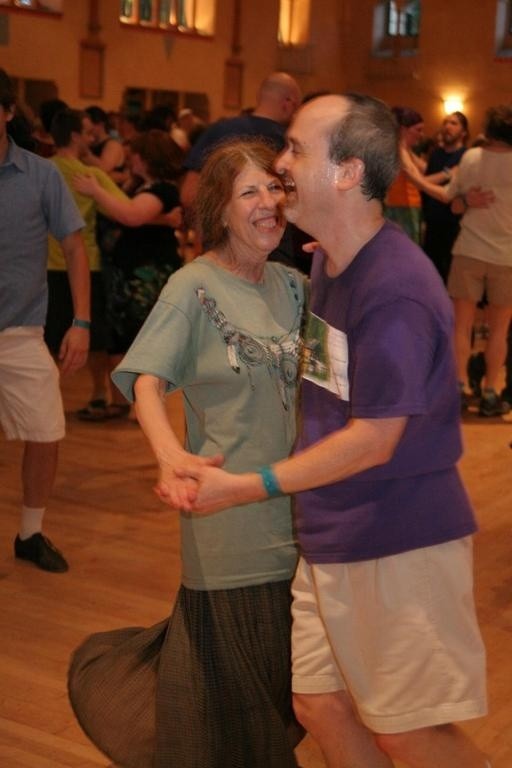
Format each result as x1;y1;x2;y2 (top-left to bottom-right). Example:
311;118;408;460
255;463;285;499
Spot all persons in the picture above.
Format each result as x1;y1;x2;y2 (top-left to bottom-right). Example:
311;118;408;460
152;89;488;768
0;71;91;573
67;140;318;768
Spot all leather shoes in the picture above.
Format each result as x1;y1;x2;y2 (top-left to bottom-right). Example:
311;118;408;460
13;532;69;573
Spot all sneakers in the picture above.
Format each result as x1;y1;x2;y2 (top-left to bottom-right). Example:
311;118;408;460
480;387;503;416
461;383;477;421
76;395;131;421
